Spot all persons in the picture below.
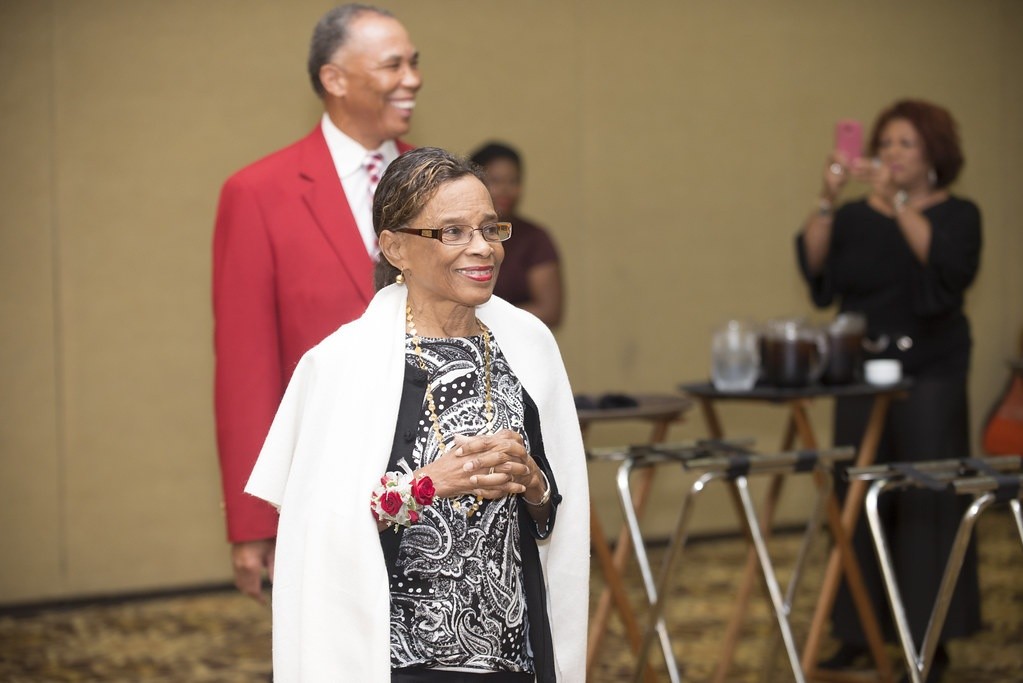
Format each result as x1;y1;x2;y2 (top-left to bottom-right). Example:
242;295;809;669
267;150;560;683
210;3;424;608
794;98;983;683
463;140;563;329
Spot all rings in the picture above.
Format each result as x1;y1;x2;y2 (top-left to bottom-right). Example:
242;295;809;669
488;463;498;478
510;473;517;483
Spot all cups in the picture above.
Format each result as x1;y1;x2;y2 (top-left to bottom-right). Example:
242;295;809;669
760;318;830;389
863;357;901;389
828;314;890;384
709;323;761;391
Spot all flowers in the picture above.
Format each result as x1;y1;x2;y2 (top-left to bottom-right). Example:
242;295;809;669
370;472;439;534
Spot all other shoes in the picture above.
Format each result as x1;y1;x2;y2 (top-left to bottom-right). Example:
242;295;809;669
816;640;874;669
901;642;950;683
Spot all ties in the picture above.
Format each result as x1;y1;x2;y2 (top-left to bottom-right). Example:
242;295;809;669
361;154;385;262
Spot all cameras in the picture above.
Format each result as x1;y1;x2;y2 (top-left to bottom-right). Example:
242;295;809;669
845;157;883;180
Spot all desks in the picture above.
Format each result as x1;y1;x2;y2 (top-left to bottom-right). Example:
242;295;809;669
575;393;697;683
677;378;915;683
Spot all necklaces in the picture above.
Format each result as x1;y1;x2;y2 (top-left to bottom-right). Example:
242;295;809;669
405;294;494;518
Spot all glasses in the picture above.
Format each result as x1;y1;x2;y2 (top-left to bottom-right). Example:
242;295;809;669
393;222;512;247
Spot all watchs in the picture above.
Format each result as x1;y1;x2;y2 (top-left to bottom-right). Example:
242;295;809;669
521;465;551;509
